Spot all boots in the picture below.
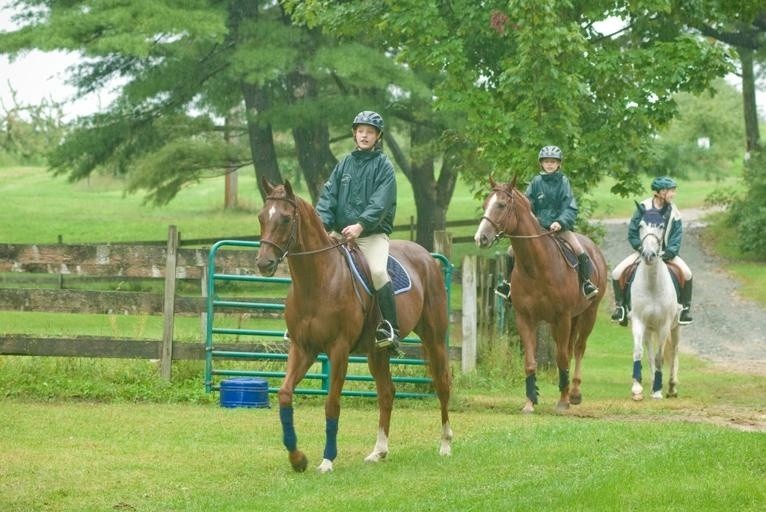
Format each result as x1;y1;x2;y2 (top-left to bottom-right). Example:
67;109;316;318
680;278;694;323
578;251;598;303
495;254;514;298
611;281;628;326
376;281;400;356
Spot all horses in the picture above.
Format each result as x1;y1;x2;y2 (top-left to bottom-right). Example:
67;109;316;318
254;174;453;474
625;199;684;402
473;175;608;414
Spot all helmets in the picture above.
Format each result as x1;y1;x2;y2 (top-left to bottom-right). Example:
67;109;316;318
352;110;384;134
537;146;563;162
651;177;678;192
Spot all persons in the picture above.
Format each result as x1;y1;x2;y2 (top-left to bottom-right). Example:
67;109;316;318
313;111;400;354
609;176;694;327
493;145;599;303
